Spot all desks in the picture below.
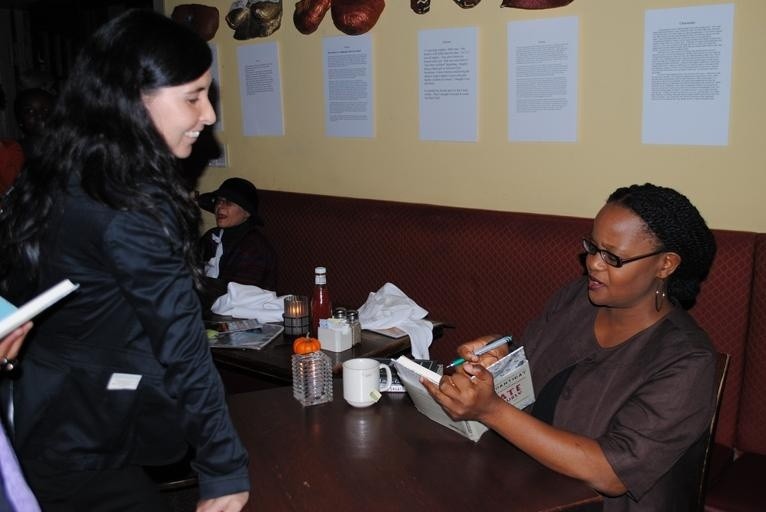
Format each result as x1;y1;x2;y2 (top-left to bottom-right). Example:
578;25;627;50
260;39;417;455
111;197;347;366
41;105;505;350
207;382;608;512
201;299;456;388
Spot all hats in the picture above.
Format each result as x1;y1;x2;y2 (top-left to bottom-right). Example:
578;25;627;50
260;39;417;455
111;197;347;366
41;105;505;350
197;177;265;228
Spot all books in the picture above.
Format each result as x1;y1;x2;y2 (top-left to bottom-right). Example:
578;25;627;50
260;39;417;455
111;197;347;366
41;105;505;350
391;345;535;443
0;277;79;339
370;357;442;392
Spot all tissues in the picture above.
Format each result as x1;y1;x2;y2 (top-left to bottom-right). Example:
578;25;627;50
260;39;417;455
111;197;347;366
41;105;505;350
318;318;353;352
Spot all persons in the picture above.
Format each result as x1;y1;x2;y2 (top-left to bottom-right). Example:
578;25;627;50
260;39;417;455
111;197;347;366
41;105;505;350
0;293;42;512
1;8;253;512
193;178;278;320
419;185;718;512
1;89;57;196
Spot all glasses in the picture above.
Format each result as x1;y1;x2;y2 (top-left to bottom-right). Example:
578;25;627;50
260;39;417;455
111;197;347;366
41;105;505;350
582;238;664;268
212;199;233;208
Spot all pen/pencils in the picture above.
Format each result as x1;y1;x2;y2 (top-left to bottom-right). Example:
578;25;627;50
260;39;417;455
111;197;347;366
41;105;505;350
446;334;513;368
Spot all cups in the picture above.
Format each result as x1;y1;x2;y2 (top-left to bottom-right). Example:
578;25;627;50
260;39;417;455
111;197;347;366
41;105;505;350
341;356;393;409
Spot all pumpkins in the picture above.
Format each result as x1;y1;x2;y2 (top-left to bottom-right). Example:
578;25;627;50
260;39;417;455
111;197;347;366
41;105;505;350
292;331;321;355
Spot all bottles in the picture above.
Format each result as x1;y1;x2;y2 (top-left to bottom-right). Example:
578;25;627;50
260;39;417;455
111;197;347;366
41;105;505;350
345;310;362;347
335;307;347;321
309;267;334;340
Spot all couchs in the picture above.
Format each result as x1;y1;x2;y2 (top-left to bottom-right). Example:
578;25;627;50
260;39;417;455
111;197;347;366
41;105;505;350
706;233;766;512
245;190;757;491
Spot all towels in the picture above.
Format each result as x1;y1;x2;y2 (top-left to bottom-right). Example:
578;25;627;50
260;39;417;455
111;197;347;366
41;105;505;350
357;282;433;360
211;281;293;325
